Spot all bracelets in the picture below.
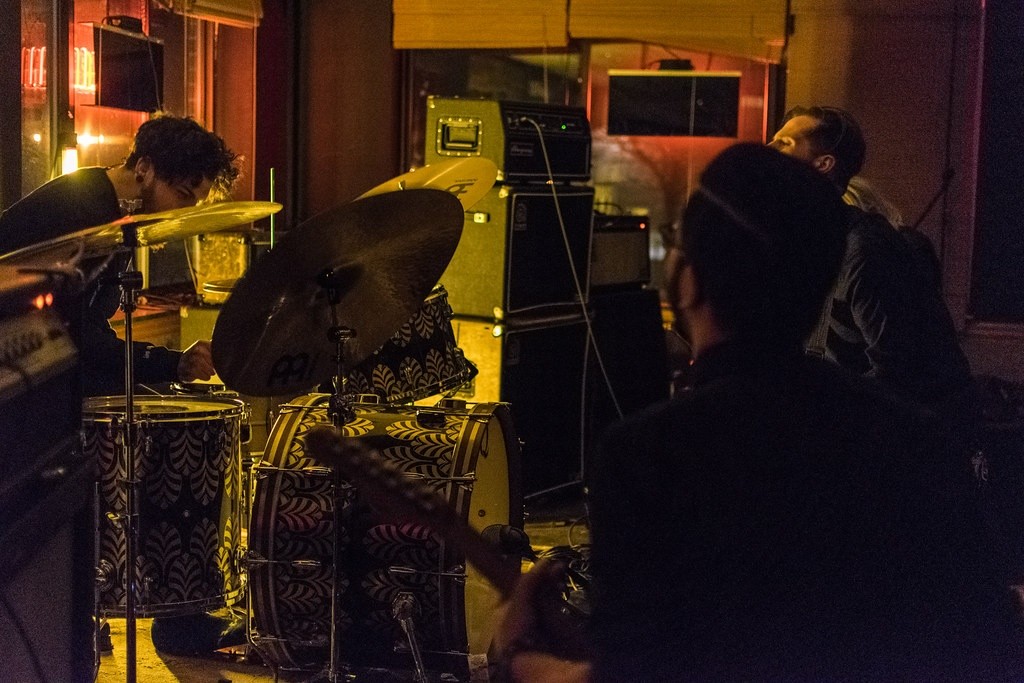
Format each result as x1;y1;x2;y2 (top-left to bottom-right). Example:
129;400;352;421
495;632;546;683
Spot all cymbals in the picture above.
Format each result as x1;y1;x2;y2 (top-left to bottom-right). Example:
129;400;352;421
1;200;283;267
350;156;499;215
210;186;465;396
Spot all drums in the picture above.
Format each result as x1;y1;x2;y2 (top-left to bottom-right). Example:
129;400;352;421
315;283;469;405
84;393;248;619
240;391;525;682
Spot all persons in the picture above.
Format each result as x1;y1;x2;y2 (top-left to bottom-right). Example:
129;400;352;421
1;114;245;402
493;99;1024;682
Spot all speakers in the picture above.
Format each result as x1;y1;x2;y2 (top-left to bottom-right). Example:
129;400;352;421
426;97;661;505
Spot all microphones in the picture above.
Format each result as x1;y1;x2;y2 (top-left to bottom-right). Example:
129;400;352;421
486;526;530;555
